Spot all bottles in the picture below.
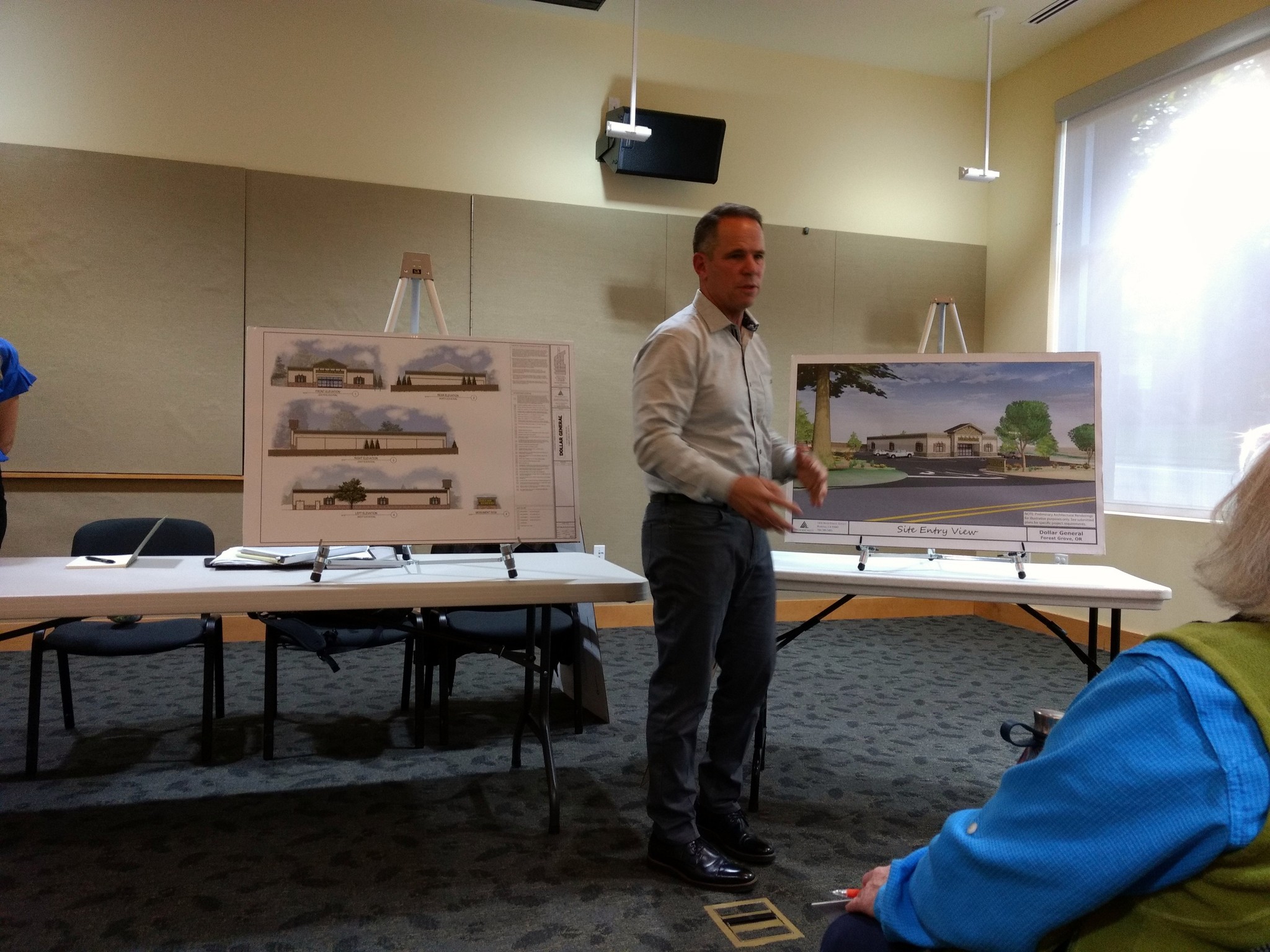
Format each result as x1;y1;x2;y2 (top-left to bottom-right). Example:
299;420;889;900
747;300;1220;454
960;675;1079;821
1000;708;1065;764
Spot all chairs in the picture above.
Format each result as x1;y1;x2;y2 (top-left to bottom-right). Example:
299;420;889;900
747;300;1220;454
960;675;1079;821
423;542;578;732
26;516;227;780
247;542;418;762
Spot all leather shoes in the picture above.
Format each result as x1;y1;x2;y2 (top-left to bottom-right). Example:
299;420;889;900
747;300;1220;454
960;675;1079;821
694;810;778;862
648;832;760;890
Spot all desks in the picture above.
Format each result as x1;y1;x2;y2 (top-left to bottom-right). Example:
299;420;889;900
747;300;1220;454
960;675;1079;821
741;551;1174;809
0;551;649;805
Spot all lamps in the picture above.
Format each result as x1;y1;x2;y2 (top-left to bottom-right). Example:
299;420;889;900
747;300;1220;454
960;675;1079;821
958;6;1004;184
606;1;652;143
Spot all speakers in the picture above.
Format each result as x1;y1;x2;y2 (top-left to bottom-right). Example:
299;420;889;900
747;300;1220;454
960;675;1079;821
601;107;726;184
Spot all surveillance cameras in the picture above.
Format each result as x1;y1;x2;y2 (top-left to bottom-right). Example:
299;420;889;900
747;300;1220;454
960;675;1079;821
606;119;652;142
959;166;1001;185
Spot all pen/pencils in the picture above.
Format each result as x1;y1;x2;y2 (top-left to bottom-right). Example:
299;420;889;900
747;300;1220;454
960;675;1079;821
830;888;861;899
86;556;117;564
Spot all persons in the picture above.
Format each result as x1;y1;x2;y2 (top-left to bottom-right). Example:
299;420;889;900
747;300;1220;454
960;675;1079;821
0;338;38;546
632;205;828;893
820;440;1270;952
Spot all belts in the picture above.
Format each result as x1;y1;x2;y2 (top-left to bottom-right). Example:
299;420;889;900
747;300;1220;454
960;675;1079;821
648;493;726;508
